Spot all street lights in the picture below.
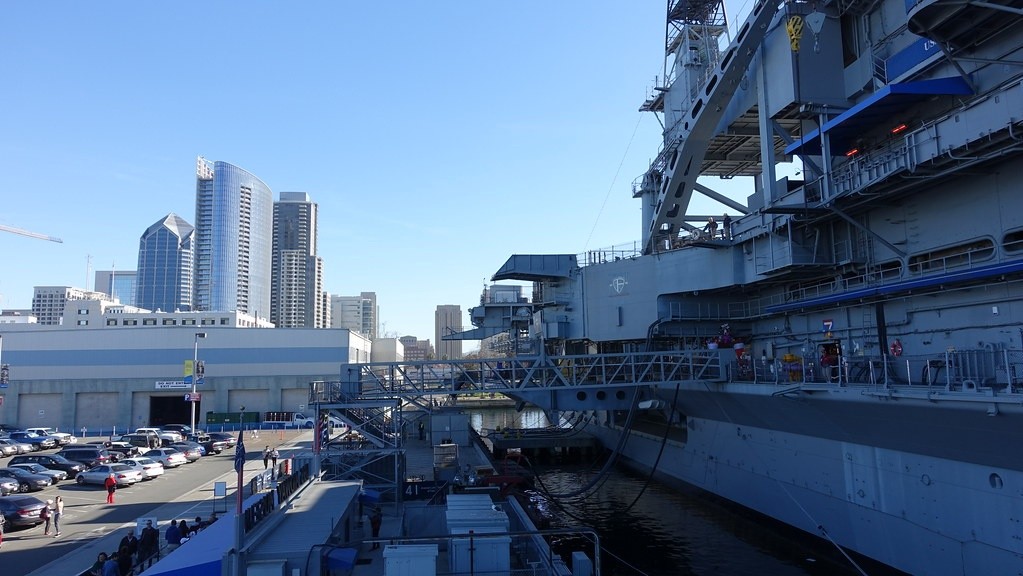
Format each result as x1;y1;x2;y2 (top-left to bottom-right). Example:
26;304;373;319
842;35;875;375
190;332;207;435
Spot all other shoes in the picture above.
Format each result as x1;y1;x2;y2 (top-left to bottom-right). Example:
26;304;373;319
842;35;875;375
46;532;52;535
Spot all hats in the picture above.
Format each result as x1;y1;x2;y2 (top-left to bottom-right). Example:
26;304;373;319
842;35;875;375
47;500;53;505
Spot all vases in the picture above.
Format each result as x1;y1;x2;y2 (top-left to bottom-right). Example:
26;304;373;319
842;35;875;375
707;344;718;350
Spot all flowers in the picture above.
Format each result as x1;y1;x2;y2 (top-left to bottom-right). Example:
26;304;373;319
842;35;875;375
706;337;718;343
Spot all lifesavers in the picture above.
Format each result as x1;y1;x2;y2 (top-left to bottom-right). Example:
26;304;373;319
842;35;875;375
501;427;511;438
890;340;903;356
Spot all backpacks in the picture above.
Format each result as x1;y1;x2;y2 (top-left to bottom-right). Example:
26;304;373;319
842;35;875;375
40;507;46;518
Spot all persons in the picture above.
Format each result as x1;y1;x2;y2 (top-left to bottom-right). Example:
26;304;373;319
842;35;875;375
204;513;219;530
262;446;271;470
54;496;64;537
190;526;197;535
197;522;206;534
118;528;138;576
90;546;133;576
44;499;53;535
178;519;191;545
820;348;834;383
189;516;201;535
0;510;6;549
197;526;197;528
137;520;158;575
105;473;116;503
360;491;384;552
165;519;184;554
703;213;733;240
270;448;279;468
417;420;424;440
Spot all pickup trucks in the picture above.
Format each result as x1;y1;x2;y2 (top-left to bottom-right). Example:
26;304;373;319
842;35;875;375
262;412;314;429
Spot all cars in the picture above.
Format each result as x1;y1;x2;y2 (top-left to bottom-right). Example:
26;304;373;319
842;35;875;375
0;423;240;531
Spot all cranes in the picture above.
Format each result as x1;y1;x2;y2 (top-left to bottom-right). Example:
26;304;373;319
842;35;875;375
0;225;63;243
